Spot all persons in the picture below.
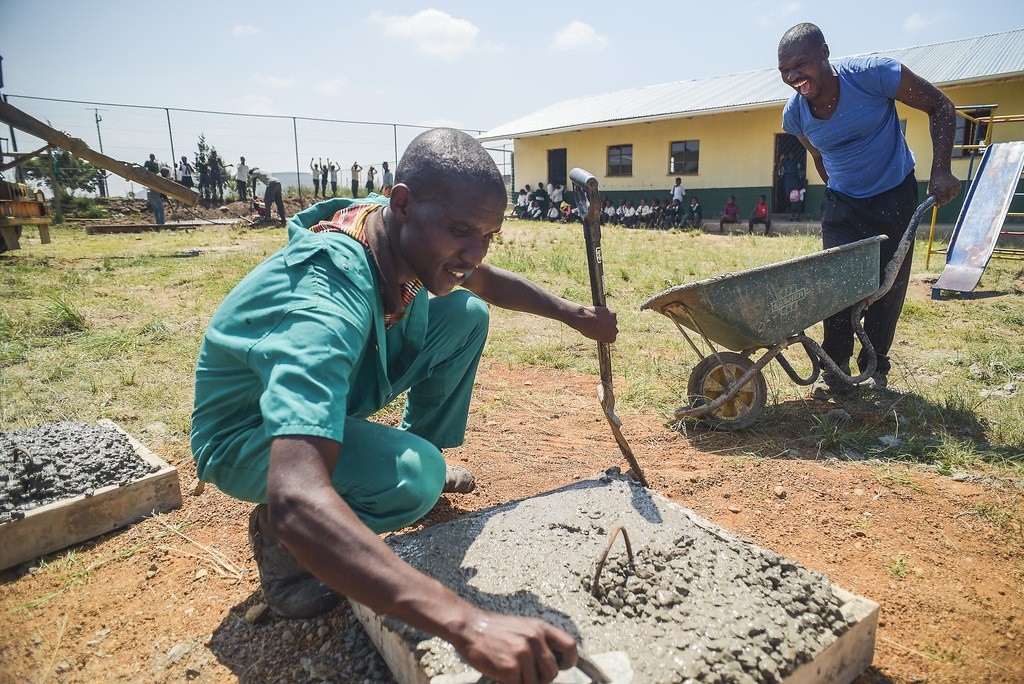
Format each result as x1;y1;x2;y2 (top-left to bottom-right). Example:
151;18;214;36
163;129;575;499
147;188;165;224
719;150;809;236
635;199;659;229
777;21;962;401
248;169;286;223
319;158;328;196
561;199;583;224
207;149;233;201
179;156;195;188
191;127;619;684
351;161;362;198
519;194;534;218
525;184;533;208
326;158;340;196
310;158;324;198
528;178;566;221
144;153;159;174
654;178;702;230
510;188;526;215
235;156;250;202
365;166;377;195
601;195;615;225
615;198;636;229
195;155;210;200
380;162;393;196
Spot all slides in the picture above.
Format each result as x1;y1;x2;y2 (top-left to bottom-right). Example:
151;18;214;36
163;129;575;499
928;141;1024;293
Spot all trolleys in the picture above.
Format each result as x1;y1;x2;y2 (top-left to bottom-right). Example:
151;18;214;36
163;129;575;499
640;193;936;430
251;198;274;218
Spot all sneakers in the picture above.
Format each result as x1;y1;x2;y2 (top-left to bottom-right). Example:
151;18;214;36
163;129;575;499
811;361;852;400
859;363;887;387
248;503;323;617
442;463;475;494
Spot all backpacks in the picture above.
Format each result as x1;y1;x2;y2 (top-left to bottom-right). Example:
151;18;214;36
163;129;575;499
790;189;799;202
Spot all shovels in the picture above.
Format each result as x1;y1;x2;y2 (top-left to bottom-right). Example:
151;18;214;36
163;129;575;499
568;167;651;489
219;207;256;226
